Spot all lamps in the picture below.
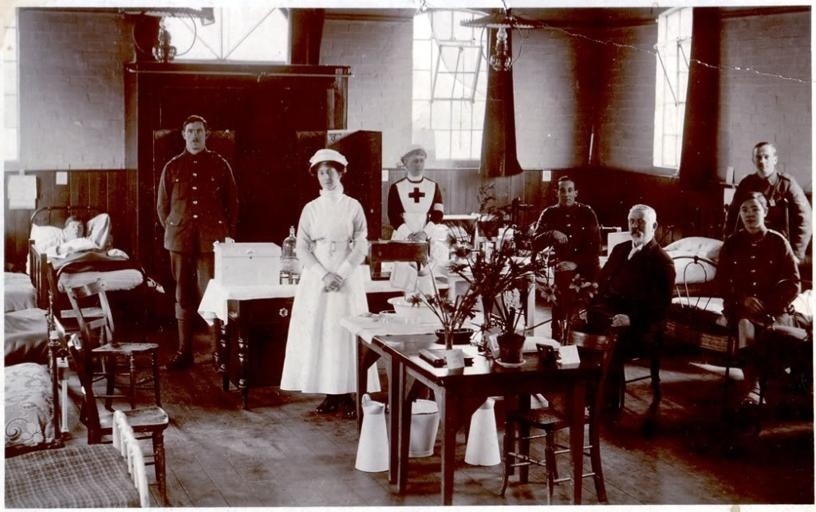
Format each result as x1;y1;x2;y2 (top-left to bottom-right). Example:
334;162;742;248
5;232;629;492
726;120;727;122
461;12;545;72
123;7;215;63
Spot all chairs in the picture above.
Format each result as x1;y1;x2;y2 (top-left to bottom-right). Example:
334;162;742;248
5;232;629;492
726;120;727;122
61;278;161;410
67;335;170;505
500;329;618;505
366;238;435;282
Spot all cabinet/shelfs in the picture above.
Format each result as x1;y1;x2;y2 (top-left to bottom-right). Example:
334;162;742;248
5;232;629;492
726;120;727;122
124;65;352;332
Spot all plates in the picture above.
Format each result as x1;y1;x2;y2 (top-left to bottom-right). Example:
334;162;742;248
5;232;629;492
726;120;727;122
436;328;475;341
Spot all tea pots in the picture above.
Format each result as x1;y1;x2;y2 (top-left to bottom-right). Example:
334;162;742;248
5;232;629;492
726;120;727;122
355;394;389;472
464;398;500;466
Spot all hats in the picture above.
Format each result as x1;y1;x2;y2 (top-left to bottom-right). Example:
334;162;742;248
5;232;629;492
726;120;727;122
309;149;348;168
400;144;426;161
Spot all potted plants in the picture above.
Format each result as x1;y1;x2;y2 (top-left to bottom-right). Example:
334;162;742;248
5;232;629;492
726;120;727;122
415;186;560;362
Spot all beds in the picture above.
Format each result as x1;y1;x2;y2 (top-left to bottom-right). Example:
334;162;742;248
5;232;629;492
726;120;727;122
5;206;147;508
598;231;812;459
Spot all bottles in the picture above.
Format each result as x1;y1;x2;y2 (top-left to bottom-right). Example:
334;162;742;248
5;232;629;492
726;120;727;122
281;272;300;285
282;226;296;257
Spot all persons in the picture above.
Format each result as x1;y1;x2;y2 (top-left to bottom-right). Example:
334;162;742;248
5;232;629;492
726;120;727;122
716;189;801;408
531;174;603;340
157;115;238;373
388;145;443;264
576;204;675;421
722;142;812;265
279;149;382;416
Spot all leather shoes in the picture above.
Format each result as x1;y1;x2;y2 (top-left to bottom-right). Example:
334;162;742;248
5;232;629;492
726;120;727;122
740;393;767;409
163;350;192;370
315;397;357;418
212;351;225;373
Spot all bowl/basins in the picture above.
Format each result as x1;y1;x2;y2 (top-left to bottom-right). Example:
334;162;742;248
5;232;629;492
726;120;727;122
387;297;435;315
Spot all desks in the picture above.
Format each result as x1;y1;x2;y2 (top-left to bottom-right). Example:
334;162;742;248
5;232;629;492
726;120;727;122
340;312;485;484
372;330;601;505
213;281;404;410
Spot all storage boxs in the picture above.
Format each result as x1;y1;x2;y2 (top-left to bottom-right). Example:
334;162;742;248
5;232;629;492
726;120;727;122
214;243;281;292
